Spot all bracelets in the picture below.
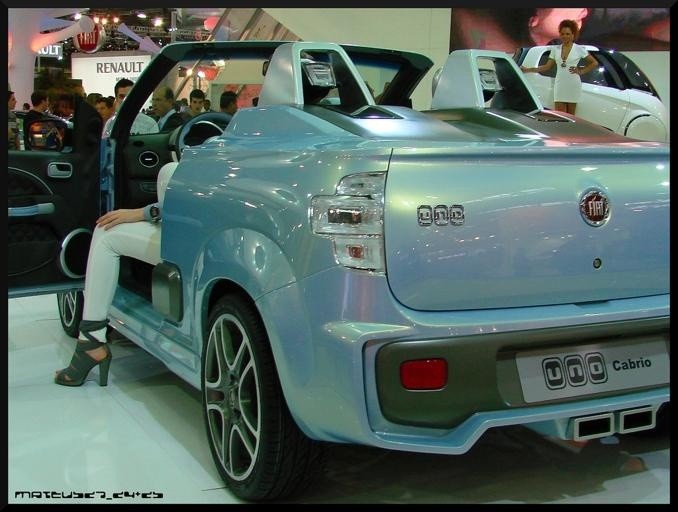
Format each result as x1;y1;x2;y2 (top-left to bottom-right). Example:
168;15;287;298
525;67;528;73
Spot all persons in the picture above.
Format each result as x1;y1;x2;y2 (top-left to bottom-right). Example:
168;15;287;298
7;79;238;148
54;162;179;387
520;19;598;115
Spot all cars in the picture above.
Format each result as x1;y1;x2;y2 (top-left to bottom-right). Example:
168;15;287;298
9;106;71;150
450;45;667;143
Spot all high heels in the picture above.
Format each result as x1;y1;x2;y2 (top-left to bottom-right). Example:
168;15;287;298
54;341;112;387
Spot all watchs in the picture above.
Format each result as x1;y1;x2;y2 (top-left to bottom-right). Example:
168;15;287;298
149;203;160;223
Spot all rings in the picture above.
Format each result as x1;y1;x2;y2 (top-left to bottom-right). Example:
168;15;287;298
573;68;575;69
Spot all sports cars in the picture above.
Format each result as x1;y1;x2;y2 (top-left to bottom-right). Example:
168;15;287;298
5;38;675;507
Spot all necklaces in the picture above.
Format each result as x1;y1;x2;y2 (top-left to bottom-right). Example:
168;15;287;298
560;46;572;67
